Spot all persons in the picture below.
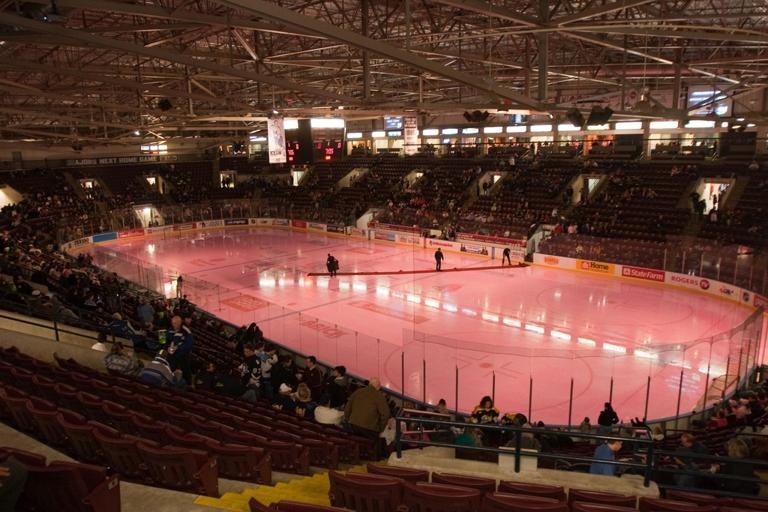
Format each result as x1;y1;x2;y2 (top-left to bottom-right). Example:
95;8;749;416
0;141;768;512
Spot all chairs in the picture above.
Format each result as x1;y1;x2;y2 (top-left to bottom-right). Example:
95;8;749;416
0;345;768;512
